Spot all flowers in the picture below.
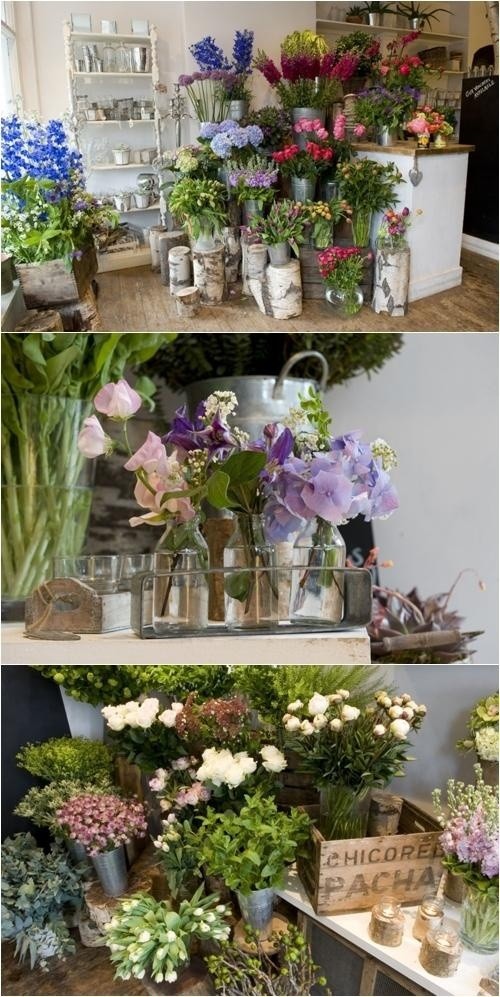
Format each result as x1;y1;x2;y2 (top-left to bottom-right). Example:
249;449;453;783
2;665;499;995
2;0;459;323
36;376;492;665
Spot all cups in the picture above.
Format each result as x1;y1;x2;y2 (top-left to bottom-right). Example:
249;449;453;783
130;46;147;73
50;554;123;597
120;553;156;590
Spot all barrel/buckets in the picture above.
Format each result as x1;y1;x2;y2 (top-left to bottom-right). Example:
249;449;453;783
91;845;133;899
182;351;332;466
226;101;246;124
292;176;315;205
240;886;273;939
295;107;326;142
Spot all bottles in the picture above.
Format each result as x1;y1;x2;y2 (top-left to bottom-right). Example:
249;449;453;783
465;65;494;76
476;960;499;996
411;896;447;939
369;896;403;948
76;93;90;113
422;916;462;978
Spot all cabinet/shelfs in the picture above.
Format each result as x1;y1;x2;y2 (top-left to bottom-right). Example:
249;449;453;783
281;134;477;310
315;2;467;114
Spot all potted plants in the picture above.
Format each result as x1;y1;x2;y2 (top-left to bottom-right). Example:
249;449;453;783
1;334;180;620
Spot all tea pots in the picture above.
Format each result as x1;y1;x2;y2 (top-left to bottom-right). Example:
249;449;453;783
328;5;346;20
